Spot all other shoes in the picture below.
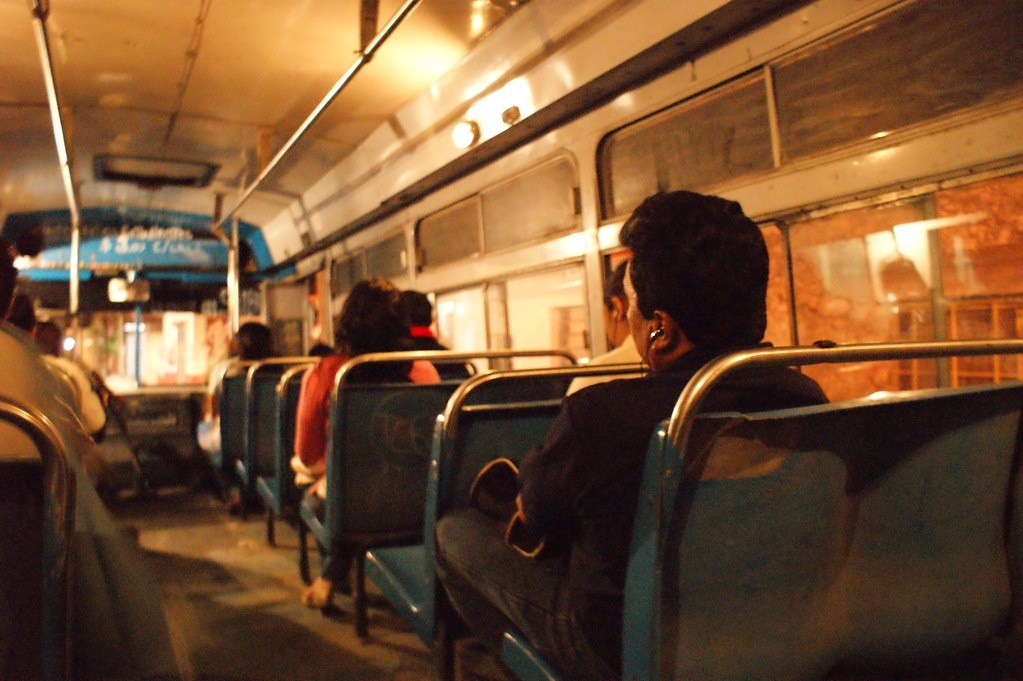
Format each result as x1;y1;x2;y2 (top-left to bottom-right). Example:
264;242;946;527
301;586;333;609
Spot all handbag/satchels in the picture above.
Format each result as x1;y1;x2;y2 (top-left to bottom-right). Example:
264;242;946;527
470;458;551;561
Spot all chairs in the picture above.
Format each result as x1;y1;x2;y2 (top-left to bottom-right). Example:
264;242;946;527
0;397;76;681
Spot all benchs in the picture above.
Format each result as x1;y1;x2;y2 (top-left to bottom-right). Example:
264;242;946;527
210;338;1023;679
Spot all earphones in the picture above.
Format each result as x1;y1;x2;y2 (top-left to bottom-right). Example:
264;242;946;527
649;323;665;345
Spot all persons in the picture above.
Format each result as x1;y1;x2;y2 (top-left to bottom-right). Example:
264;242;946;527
290;277;473;611
197;322;282;518
566;259;649;401
0;251;126;681
437;190;830;681
